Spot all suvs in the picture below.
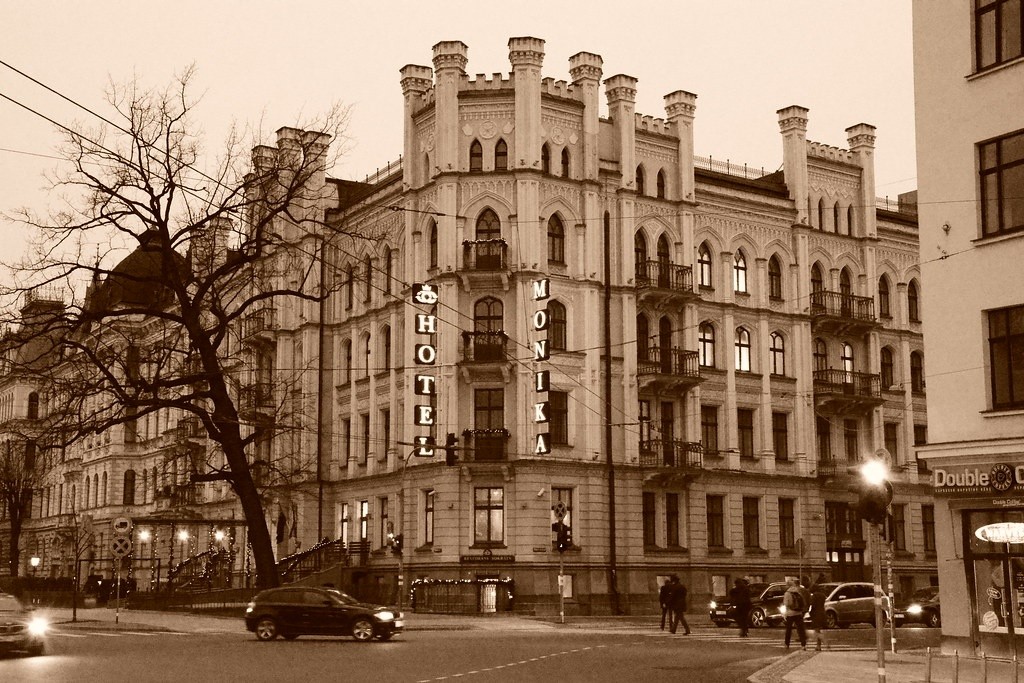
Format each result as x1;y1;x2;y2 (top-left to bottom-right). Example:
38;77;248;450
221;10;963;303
804;582;890;628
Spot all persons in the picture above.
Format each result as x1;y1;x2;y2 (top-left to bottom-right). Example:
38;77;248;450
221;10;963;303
667;573;691;636
808;583;832;651
659;579;674;632
728;579;753;639
781;575;808;651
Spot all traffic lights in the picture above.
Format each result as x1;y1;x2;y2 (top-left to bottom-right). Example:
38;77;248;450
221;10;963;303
847;464;873;516
556;524;573;552
880;514;894;544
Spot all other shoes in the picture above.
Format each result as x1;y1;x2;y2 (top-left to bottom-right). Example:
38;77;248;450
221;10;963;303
739;630;748;638
782;645;789;651
683;632;690;635
802;647;807;651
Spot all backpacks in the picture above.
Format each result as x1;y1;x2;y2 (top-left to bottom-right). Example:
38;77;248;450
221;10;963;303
786;589;805;612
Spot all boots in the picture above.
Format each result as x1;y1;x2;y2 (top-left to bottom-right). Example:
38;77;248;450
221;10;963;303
814;638;821;651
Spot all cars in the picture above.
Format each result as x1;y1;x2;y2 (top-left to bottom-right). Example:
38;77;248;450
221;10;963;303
891;587;940;627
245;587;406;639
710;581;803;628
0;595;43;653
